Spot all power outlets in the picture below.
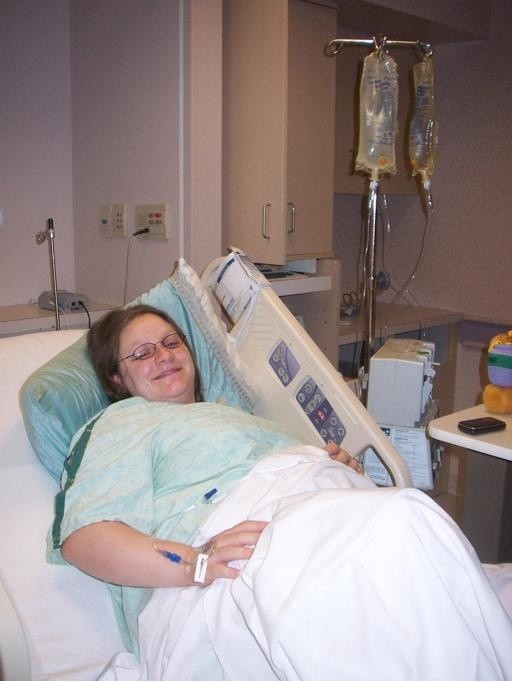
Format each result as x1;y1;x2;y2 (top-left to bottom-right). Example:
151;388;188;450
110;205;127;240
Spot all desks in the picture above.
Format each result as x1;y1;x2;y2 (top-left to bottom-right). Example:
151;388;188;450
426;402;511;465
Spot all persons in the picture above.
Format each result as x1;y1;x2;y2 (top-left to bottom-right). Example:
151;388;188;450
52;304;366;658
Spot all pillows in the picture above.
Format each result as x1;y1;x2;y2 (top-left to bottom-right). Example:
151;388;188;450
17;256;259;491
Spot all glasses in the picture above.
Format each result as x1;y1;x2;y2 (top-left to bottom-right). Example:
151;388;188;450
114;334;187;366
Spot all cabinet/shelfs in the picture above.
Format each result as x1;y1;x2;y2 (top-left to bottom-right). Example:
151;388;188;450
323;298;465;503
222;0;339;371
0;297;120;338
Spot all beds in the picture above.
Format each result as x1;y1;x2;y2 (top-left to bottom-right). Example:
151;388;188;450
0;260;512;681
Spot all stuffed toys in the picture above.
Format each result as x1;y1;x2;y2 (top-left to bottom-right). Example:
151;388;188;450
482;330;512;414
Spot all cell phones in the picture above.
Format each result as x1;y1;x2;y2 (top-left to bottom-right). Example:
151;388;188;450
459;417;507;435
